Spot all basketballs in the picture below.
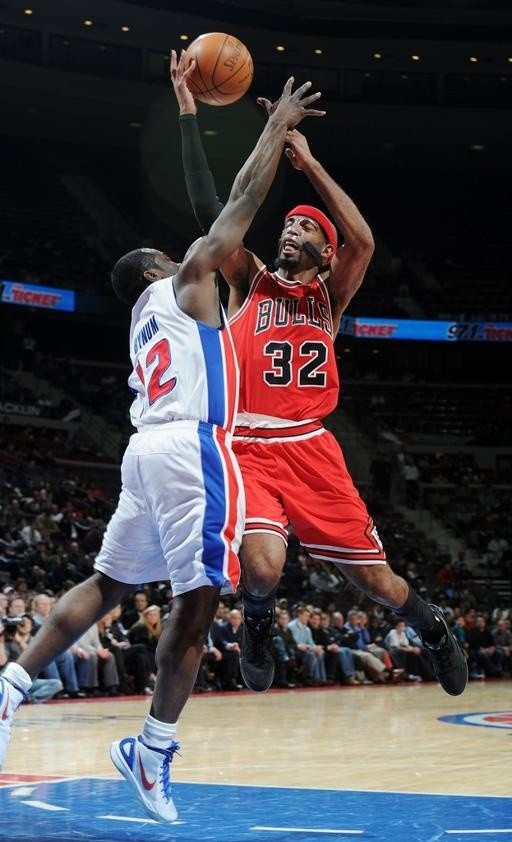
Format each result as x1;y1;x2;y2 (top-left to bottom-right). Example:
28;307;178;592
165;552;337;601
182;33;254;107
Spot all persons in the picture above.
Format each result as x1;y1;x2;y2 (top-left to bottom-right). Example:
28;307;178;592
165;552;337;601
0;301;512;709
165;46;470;700
0;70;334;826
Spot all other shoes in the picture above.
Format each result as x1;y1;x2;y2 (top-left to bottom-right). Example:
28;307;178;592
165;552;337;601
55;676;420;693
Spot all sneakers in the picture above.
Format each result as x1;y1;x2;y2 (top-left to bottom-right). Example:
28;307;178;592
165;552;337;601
111;737;179;824
0;676;27;770
421;603;468;696
240;585;277;691
